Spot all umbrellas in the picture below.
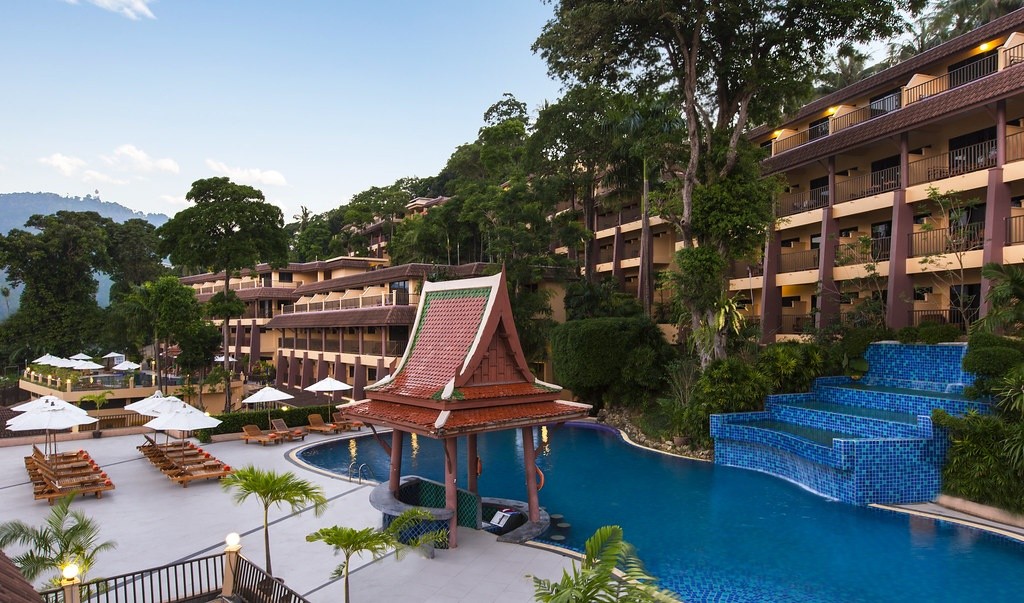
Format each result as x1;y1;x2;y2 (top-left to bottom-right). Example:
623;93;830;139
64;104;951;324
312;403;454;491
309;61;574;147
303;375;354;423
5;392;98;482
241;384;295;429
33;352;141;375
124;389;223;474
215;355;238;362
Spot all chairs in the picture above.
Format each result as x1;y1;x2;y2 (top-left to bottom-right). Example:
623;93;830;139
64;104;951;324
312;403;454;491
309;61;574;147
306;414;344;434
242;425;281;446
271;419;309;441
136;434;236;488
24;442;115;506
332;412;365;431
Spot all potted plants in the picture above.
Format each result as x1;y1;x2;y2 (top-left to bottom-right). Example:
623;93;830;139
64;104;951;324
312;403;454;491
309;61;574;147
666;402;692;446
219;464;327;597
79;391;115;438
